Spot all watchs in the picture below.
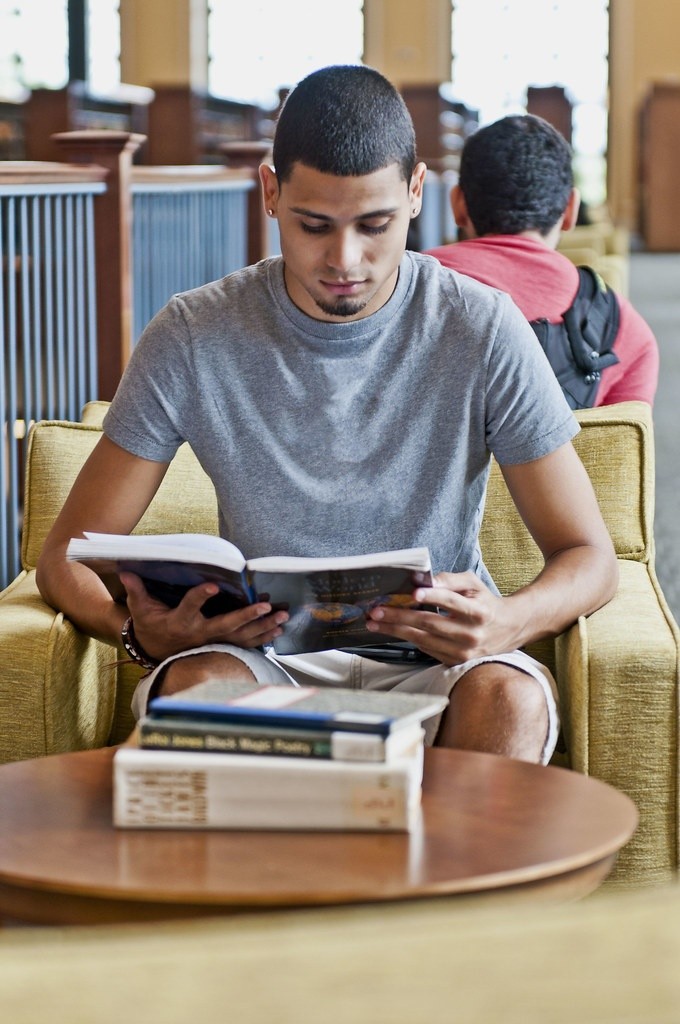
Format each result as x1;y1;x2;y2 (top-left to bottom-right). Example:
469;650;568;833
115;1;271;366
122;615;156;671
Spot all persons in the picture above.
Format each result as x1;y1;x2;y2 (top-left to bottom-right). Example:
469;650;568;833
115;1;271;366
35;66;619;766
417;114;659;410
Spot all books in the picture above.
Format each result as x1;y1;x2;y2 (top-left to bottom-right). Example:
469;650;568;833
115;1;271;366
113;679;449;833
66;531;439;657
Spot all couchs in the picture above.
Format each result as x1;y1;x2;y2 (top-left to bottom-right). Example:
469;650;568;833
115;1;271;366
1;400;680;891
559;203;630;303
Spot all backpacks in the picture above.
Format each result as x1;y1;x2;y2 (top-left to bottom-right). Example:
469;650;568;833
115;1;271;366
523;264;620;412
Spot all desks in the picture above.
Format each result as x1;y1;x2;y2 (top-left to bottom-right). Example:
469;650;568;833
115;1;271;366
0;740;639;929
